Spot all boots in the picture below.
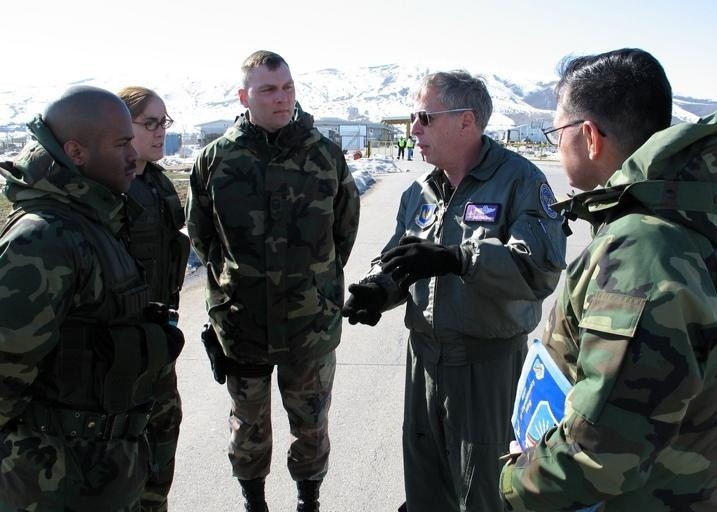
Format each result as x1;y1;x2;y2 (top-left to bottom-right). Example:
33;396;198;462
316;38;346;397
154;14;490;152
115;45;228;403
239;479;267;511
296;480;320;511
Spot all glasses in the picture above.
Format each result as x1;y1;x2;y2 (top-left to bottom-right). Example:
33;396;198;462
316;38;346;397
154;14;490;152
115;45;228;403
130;112;175;132
409;107;474;128
540;120;607;146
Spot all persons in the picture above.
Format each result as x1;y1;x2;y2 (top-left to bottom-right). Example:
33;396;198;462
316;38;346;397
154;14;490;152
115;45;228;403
183;51;356;511
115;87;191;503
499;48;717;512
406;136;414;161
397;136;406;160
341;70;573;512
0;86;168;503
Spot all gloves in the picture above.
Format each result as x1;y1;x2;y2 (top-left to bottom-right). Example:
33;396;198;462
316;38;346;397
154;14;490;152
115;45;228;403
381;236;461;290
341;282;387;327
